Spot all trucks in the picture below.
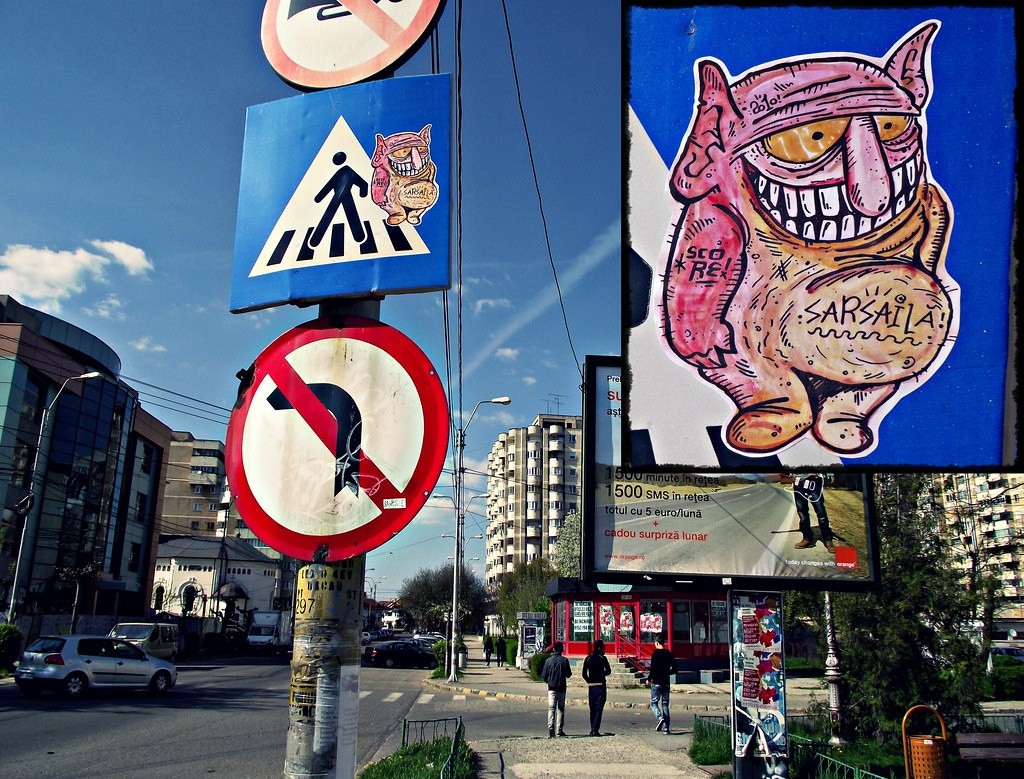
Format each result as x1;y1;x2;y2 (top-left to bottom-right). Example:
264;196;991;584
244;610;292;655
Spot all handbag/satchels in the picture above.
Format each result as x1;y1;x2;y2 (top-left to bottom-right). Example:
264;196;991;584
483;653;486;659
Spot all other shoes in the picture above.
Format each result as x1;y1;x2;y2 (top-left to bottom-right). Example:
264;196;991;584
656;718;665;731
590;731;601;737
664;731;669;735
557;731;567;736
795;538;815;548
825;540;836;553
548;729;555;738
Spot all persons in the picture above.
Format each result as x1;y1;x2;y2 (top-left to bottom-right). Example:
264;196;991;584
542;641;572;737
776;473;837;554
495;636;507;667
645;635;676;735
582;640;611;735
413;631;420;639
483;637;494;668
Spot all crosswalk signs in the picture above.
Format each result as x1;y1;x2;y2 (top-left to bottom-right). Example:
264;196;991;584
227;68;456;317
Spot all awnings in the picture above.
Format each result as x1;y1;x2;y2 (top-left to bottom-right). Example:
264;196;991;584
214;583;248;598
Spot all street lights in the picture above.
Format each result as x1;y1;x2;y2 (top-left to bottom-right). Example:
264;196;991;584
440;393;513;685
364;580;382;628
431;491;492;638
6;371;104;627
366;576;387;630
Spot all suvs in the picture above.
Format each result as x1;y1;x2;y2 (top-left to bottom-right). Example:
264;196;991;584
100;622;180;665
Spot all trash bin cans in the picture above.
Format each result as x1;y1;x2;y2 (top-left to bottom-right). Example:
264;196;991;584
902;705;948;779
457;645;466;668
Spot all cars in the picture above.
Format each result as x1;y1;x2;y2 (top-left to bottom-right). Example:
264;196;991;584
13;631;180;705
364;641;438;670
361;627;450;653
